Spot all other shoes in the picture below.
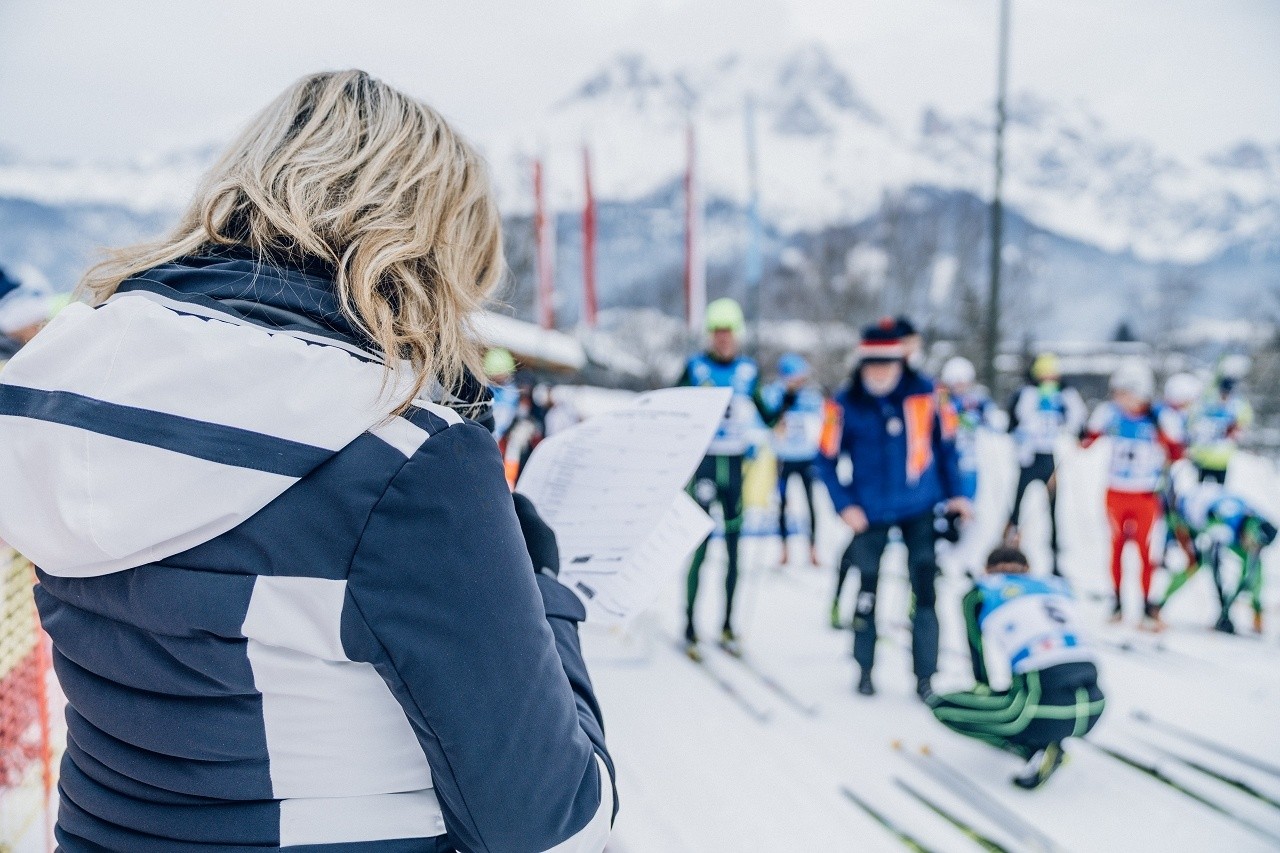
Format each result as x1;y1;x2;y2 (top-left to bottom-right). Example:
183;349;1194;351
687;642;702;662
917;682;937;704
859;677;873;693
721;633;740;656
1107;601;1241;636
1013;738;1068;791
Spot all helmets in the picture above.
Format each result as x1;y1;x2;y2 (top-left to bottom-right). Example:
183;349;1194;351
1111;362;1153;398
941;355;973;386
1032;351;1061;378
705;297;746;342
483;348;516;374
1165;376;1197;402
1223;354;1250;377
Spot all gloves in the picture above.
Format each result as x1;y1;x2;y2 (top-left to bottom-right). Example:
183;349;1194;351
508;491;560;582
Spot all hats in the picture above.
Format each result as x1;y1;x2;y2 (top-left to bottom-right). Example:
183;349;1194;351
858;318;909;356
776;354;807;375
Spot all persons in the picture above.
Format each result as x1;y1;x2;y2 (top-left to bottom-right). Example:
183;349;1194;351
0;68;620;853
670;298;1279;790
479;348;554;492
1;283;51;367
